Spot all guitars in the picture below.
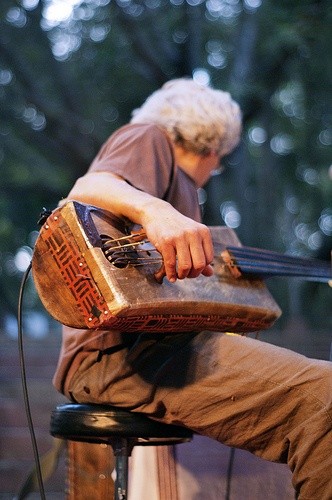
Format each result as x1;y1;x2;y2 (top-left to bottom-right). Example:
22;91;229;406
31;200;332;332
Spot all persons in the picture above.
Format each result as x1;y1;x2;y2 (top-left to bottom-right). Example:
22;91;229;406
53;78;332;500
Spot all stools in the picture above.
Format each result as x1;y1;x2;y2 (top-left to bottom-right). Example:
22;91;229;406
48;402;194;500
